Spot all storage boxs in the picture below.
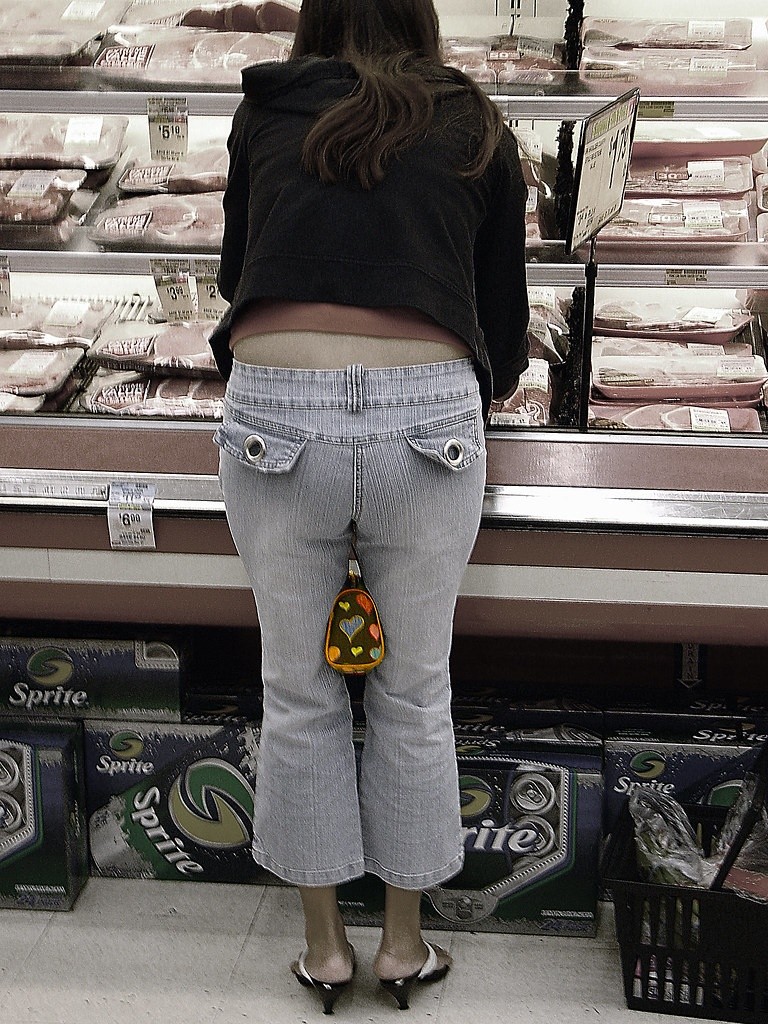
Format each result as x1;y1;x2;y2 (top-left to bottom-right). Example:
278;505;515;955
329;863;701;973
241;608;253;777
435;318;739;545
0;639;768;1024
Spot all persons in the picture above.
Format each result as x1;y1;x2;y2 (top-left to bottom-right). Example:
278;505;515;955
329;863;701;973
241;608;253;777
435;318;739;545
206;0;532;1017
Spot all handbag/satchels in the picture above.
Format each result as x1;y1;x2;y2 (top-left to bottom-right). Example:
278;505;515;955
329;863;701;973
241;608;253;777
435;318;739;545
326;533;385;674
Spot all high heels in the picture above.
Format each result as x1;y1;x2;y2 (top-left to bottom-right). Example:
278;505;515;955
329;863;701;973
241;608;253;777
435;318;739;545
380;940;452;1010
290;941;355;1015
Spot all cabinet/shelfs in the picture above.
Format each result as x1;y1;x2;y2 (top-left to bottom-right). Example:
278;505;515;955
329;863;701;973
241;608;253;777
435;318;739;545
0;90;768;650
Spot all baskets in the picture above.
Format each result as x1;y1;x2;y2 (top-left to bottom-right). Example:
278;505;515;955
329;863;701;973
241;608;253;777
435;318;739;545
595;739;768;1024
